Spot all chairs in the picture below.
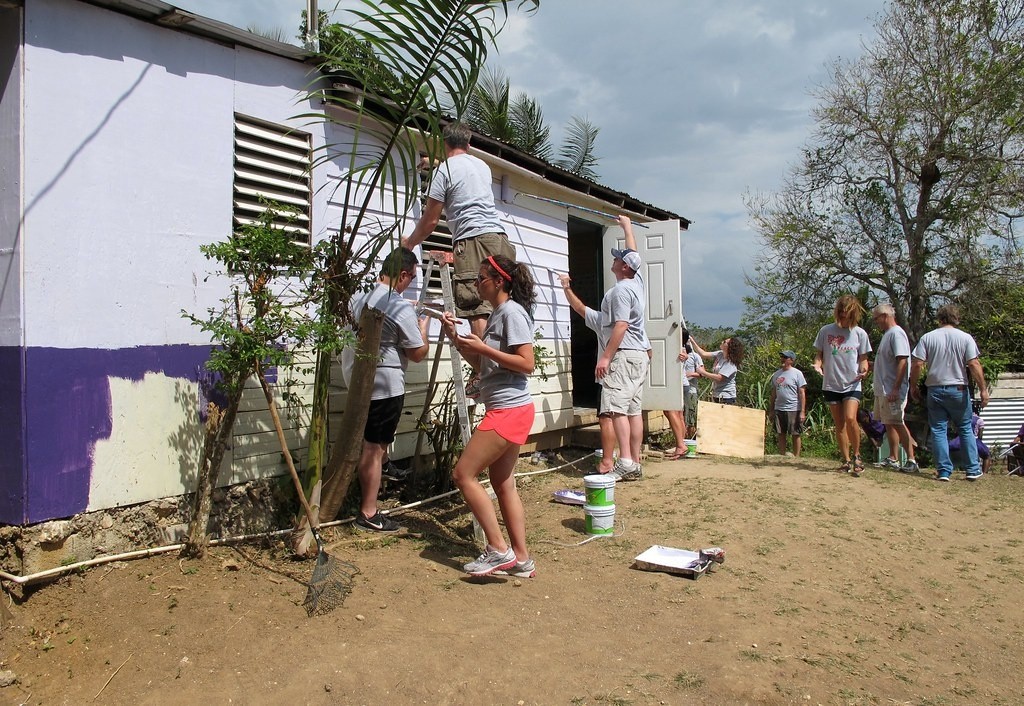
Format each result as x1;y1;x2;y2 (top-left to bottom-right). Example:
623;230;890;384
998;441;1024;477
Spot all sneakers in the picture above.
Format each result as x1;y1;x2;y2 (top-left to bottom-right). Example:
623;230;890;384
603;458;643;482
966;469;983;479
937;470;950;482
491;559;536;578
899;460;920;474
463;544;518;577
354;509;399;533
872;457;901;470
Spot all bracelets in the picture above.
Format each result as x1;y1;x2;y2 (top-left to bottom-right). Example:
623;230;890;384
457;348;461;352
563;287;572;292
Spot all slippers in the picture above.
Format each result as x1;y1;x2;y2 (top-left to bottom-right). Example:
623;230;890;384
583;466;609;475
669;447;690;461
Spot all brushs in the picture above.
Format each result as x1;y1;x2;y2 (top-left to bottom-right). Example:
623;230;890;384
547;267;572;280
423;308;463;325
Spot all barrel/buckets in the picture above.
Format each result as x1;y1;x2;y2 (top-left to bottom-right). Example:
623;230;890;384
594;449;617;466
583;505;616;537
583;474;616;507
683;439;697;458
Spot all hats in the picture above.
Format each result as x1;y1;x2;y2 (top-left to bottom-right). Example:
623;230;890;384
779;350;796;359
611;248;641;271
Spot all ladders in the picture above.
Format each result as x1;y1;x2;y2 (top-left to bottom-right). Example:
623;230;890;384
410;251;517;554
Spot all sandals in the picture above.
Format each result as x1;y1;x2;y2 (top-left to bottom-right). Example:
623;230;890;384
852;454;865;473
837;460;853;473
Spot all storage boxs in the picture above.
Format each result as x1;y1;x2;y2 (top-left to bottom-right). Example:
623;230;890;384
633;544;714;581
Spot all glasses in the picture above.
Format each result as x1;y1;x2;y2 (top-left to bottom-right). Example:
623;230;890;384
872;313;884;322
477;275;493;285
408;274;416;282
724;340;728;346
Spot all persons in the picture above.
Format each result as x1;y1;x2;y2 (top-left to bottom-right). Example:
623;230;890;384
688;334;744;405
813;295;872;473
873;304;919;473
1013;423;1024;442
769;350;807;457
948;406;991;474
557;215;652;482
911;306;989;482
399;123;517;398
663;328;705;461
342;247;429;532
453;255;537;578
857;407;886;463
